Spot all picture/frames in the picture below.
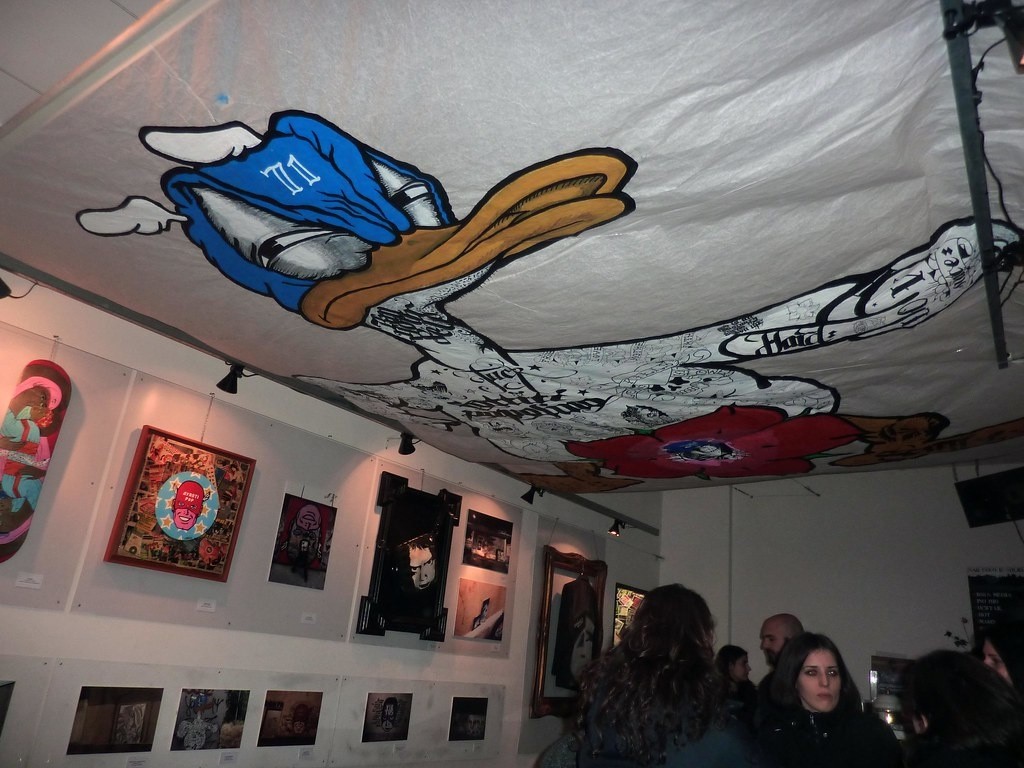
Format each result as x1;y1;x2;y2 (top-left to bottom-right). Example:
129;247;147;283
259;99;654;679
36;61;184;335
529;545;608;719
103;424;257;582
612;582;651;650
356;471;468;643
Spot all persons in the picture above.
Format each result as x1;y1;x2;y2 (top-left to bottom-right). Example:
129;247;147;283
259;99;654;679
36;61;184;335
532;583;750;768
758;613;804;707
982;633;1023;698
745;631;902;768
714;644;758;704
899;648;1024;768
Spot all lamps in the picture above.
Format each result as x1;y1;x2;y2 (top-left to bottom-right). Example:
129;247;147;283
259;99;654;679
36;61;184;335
216;363;244;394
398;433;415;455
520;484;543;505
607;518;625;537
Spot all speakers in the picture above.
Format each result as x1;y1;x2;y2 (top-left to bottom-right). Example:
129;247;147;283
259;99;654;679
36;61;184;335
955;466;1024;528
